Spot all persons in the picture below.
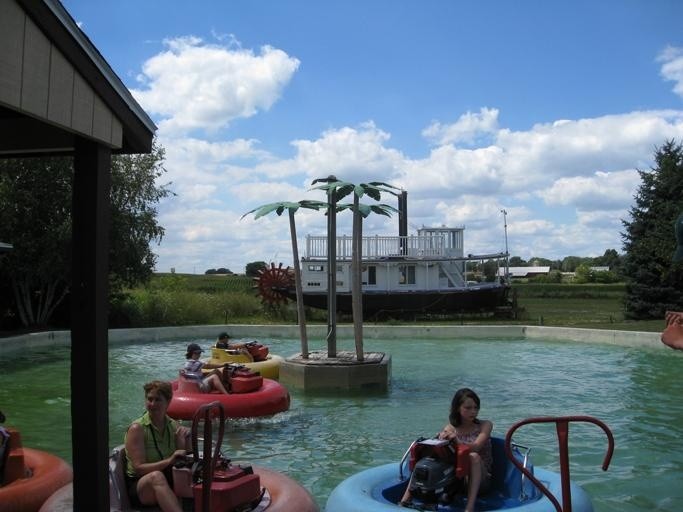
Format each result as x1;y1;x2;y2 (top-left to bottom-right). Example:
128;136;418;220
184;344;233;395
397;388;495;512
216;331;246;355
124;380;191;512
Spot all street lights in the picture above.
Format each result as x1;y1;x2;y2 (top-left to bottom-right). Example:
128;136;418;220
500;209;510;288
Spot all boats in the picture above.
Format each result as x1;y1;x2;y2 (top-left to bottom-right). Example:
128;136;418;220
323;434;591;512
38;441;321;512
195;352;284;380
0;426;72;512
251;226;512;322
157;373;289;421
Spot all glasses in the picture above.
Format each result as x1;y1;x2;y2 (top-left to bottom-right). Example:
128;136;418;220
167;462;193;469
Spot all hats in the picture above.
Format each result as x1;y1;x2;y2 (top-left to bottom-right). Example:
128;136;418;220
219;333;229;339
187;344;204;353
